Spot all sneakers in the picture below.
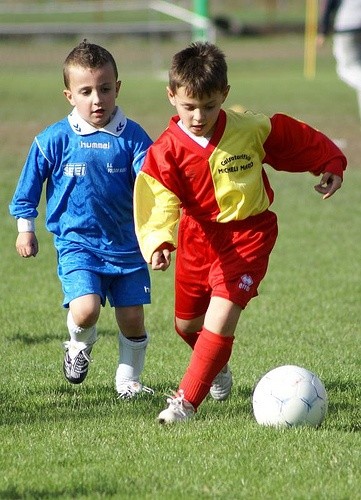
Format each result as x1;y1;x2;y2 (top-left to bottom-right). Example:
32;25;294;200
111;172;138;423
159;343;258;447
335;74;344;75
158;388;195;425
115;377;153;399
210;362;232;400
63;336;96;384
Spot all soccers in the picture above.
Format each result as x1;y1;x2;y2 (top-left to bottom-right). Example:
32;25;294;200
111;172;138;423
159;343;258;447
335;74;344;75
252;364;330;428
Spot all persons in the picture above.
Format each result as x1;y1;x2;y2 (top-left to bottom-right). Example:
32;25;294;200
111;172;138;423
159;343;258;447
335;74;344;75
9;38;154;397
133;41;347;424
